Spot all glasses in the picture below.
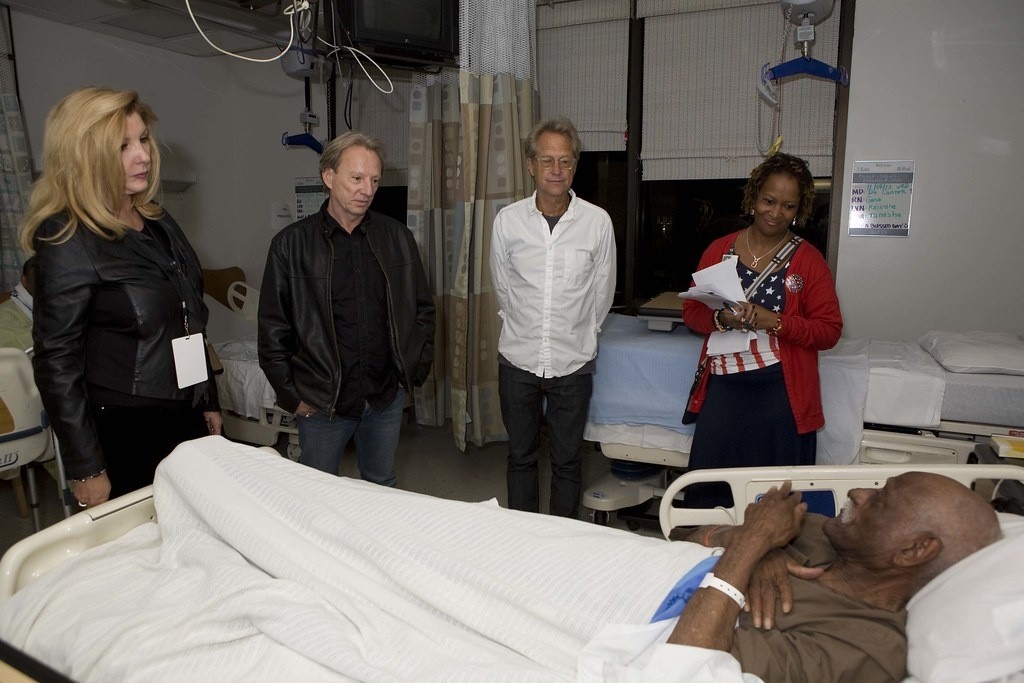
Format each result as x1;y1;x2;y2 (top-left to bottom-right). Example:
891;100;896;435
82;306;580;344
532;157;578;170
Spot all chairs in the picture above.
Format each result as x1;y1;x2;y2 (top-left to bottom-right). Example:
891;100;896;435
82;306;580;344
0;347;73;533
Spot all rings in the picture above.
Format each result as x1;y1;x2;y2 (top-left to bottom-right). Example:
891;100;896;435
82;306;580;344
305;413;309;417
77;500;87;507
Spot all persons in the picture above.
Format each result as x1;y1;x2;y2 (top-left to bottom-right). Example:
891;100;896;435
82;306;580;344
683;151;843;510
488;114;617;521
256;133;437;488
665;472;1002;683
18;85;225;509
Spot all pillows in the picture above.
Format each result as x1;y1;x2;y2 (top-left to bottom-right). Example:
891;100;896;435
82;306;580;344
904;509;1024;681
919;330;1024;375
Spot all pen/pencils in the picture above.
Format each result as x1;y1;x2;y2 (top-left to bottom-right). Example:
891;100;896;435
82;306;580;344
722;302;756;332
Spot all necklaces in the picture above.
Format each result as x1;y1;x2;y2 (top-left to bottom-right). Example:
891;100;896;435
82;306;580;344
747;224;790;269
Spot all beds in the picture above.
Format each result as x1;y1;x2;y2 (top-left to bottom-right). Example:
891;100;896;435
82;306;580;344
201;281;302;460
585;314;1024;511
1;462;1024;682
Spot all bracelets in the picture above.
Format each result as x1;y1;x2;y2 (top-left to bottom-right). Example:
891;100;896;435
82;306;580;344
698;573;745;609
713;307;732;334
766;313;781;336
704;525;721;547
66;469;105;493
